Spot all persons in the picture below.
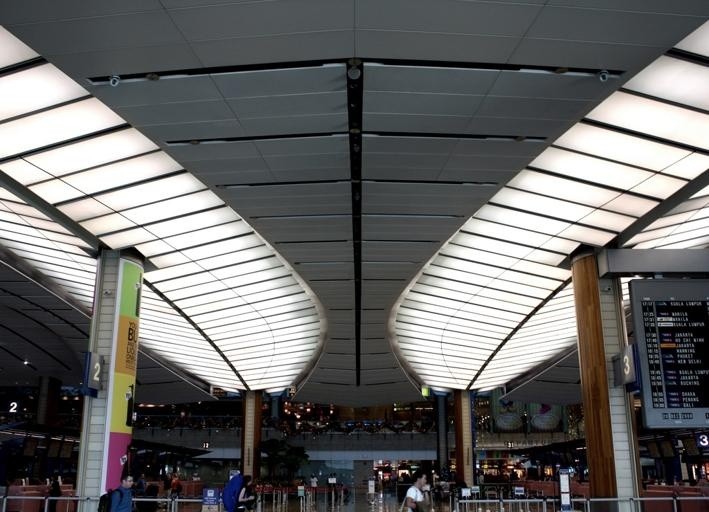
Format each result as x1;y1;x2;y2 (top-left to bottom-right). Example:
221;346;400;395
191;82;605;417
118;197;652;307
48;471;63;511
163;472;172;496
403;470;434;512
170;472;179;497
136;472;146;492
234;475;256;512
309;472;320;501
110;471;133;511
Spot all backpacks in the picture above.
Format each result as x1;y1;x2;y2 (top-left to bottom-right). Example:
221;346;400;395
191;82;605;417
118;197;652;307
98;488;123;512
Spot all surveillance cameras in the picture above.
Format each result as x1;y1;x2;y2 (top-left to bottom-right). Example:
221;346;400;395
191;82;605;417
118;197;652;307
599;71;609;82
605;287;611;291
105;290;111;295
110;75;120;87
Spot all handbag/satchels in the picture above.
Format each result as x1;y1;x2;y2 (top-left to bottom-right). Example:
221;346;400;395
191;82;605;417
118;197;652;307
398;507;413;512
